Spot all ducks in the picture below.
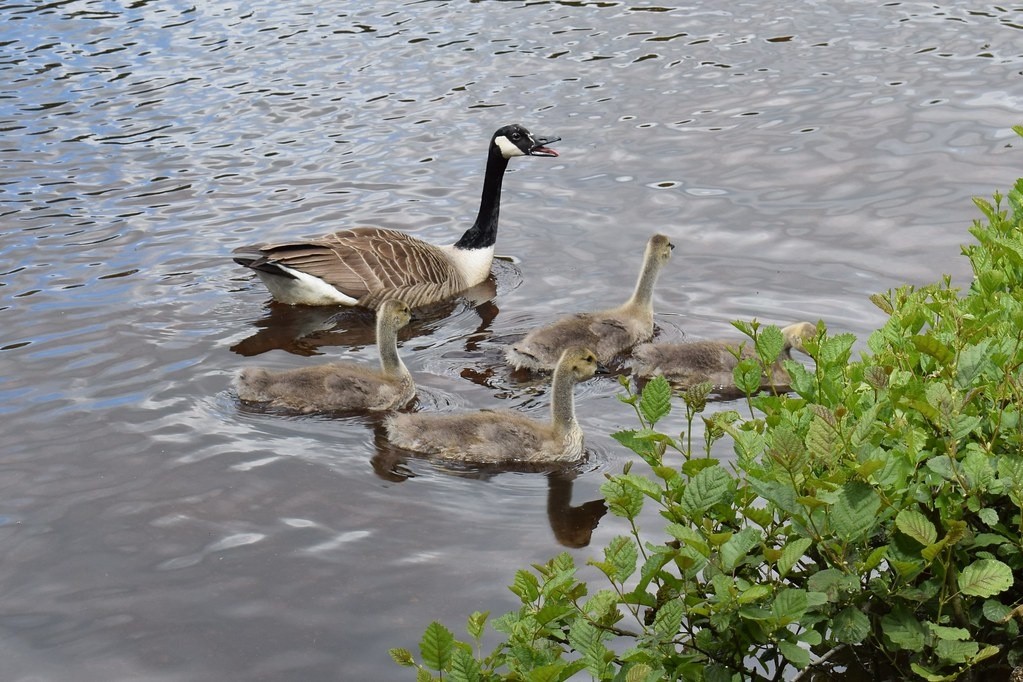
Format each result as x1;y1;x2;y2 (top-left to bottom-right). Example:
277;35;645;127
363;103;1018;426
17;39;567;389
232;125;562;314
226;235;819;469
